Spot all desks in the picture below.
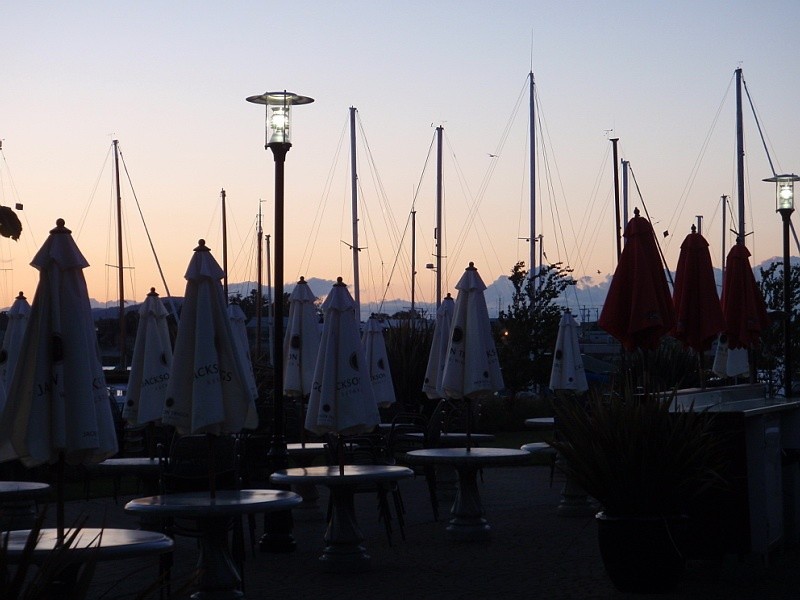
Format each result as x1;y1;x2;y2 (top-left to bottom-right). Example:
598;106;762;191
0;480;51;530
525;416;566;485
0;527;173;600
125;487;304;598
84;458;169;504
406;447;531;541
268;464;414;541
284;441;358;463
404;430;497;448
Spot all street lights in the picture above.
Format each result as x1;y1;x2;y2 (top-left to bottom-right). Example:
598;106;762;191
245;89;315;554
761;173;799;460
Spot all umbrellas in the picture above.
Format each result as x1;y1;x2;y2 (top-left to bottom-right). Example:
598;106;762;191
713;331;752;385
422;294;456;433
305;278;381;468
358;312;396;408
228;296;253;363
673;224;724;386
598;208;677;353
121;288;174;463
164;240;259;498
283;275;322;444
442;263;504;435
720;236;770;346
549;308;589;395
0;220;118;541
0;290;32;415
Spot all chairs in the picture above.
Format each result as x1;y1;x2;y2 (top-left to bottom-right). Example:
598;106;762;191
0;397;446;600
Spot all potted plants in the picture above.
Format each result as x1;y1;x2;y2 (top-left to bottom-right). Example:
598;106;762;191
543;369;743;594
